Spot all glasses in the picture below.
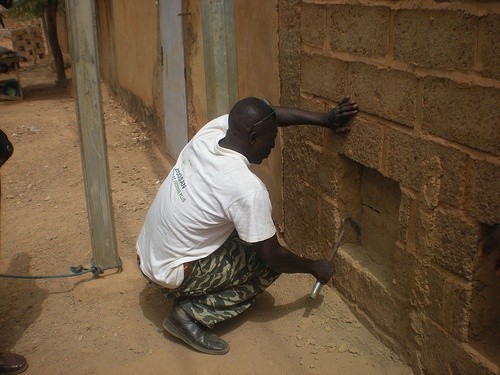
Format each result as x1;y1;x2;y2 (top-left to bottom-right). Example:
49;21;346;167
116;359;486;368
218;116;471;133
249;107;277;134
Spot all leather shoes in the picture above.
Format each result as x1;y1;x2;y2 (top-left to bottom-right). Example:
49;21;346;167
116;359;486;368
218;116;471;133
0;349;29;375
162;309;229;355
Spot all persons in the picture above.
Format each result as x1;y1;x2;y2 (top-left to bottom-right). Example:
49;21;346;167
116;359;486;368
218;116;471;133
135;95;359;355
0;127;28;375
